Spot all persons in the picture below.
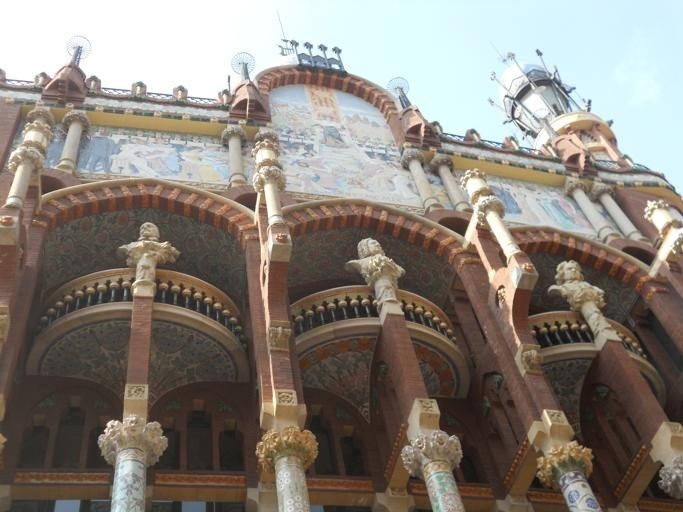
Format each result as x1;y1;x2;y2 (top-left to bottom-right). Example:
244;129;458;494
118;222;181;267
548;260;607;311
344;237;406;287
